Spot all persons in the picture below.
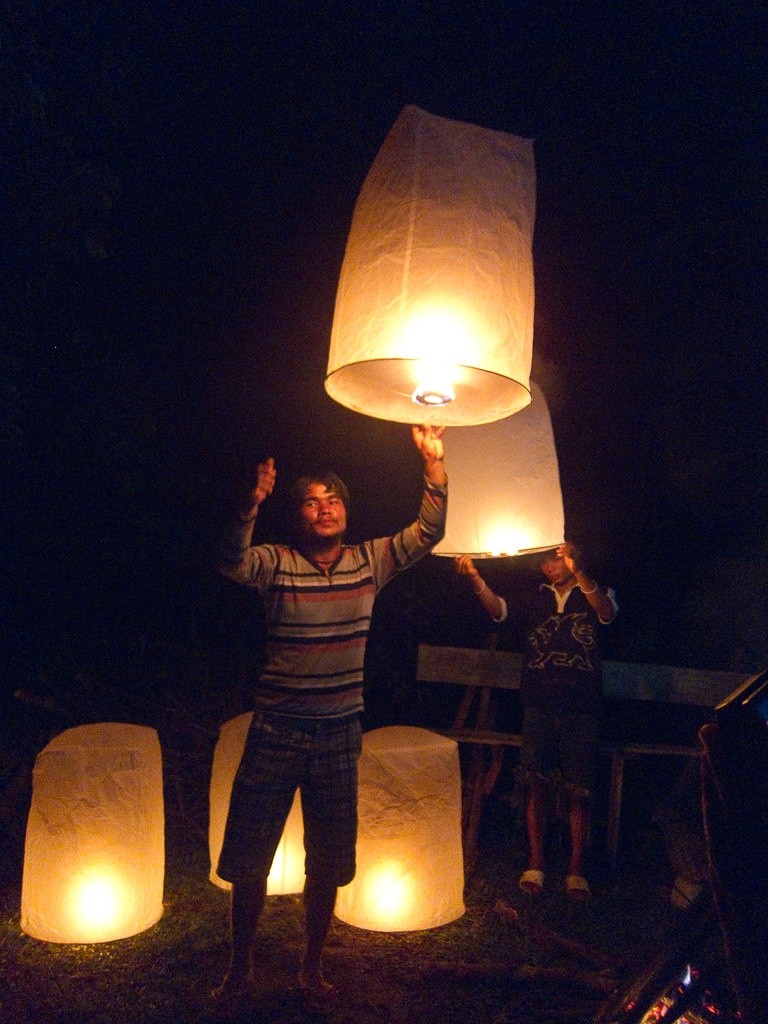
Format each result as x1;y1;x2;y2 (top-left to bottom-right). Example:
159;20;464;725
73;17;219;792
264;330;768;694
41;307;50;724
218;425;446;1004
456;543;620;906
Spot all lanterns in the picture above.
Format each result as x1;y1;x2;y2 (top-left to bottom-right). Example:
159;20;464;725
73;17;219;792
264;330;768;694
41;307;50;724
207;713;308;897
322;103;538;426
328;727;469;930
428;379;568;562
21;720;168;947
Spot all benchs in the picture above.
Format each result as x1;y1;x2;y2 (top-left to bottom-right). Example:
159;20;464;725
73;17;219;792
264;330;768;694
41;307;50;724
411;640;768;886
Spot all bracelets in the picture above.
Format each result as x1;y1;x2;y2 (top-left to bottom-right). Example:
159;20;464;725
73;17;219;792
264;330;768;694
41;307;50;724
580;578;599;595
473;580;487;595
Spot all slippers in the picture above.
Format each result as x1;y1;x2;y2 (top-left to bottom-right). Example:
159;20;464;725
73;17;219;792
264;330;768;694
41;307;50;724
520;869;544;897
566;875;591;901
208;983;255;1021
299;970;339;1014
